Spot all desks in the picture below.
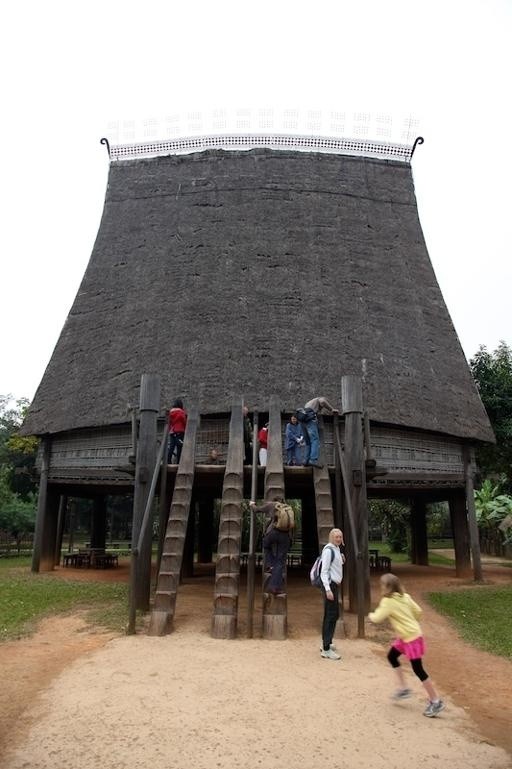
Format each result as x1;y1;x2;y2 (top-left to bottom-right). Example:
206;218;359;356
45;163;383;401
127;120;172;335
63;548;118;569
369;550;380;572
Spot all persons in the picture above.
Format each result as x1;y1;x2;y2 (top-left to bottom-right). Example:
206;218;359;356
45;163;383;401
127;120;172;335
164;399;188;463
317;528;349;661
240;407;254;465
203;450;221;465
248;497;295;596
282;413;306;468
366;573;447;719
301;396;338;469
259;423;273;468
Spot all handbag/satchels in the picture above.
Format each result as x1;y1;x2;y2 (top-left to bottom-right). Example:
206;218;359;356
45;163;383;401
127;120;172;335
175;434;184;445
294;407;316;422
311;557;322;585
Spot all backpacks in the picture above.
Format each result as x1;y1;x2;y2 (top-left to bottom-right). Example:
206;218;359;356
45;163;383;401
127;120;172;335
274;501;295;530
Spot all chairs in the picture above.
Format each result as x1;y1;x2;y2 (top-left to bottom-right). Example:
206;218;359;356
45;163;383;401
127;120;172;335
369;557;392;576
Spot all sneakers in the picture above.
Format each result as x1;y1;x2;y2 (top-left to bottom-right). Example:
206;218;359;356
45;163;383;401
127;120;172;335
263;573;271;587
320;644;340;659
423;699;445;717
308;459;323;469
394;689;409;698
